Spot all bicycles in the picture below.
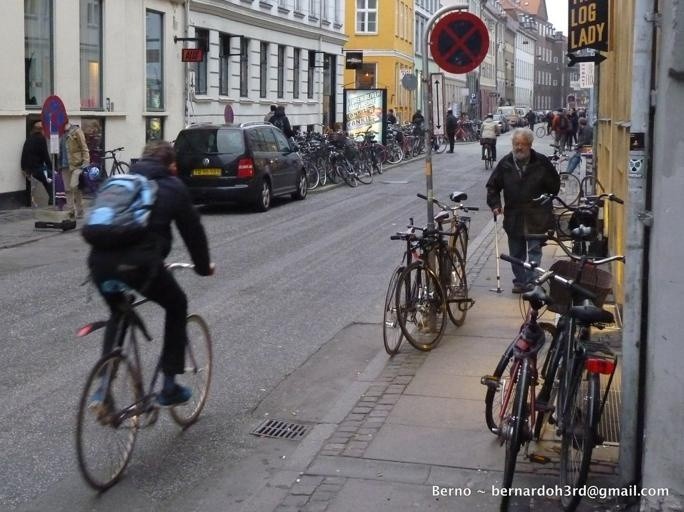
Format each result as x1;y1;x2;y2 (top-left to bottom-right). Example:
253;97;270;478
416;191;480;315
287;119;482;190
395;225;467;352
80;147;131;199
382;218;448;356
533;192;624;255
523;231;625;512
479;135;500;170
548;144;582;209
480;253;596;512
75;262;216;491
536;118;556;139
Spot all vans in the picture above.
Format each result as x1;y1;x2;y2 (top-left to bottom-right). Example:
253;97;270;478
515;108;531;127
495;106;521;128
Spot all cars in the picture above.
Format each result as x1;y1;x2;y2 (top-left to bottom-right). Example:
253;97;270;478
174;120;308;212
531;107;587;124
477;114;510;136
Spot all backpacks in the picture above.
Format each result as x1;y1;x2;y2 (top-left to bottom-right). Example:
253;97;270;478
81;171;171;248
272;117;287;132
556;115;568;131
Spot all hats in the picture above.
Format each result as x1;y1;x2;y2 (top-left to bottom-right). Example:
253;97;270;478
89;167;100;178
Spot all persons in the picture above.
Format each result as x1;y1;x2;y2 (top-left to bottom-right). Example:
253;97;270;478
55;119;90;219
263;105;276;125
562;116;593;180
483;127;561;295
386;109;396;125
445;108;457;154
479;113;500;161
411;108;423;126
19;121;53;207
525;108;535;131
269;106;290;133
85;139;212;416
540;104;593;157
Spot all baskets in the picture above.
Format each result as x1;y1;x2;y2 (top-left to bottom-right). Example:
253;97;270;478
546;260;613;315
553;204;600;242
328;133;343;143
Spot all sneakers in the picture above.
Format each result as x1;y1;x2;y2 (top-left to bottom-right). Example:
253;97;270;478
87;390;116;423
512;285;524;293
159;384;193;410
